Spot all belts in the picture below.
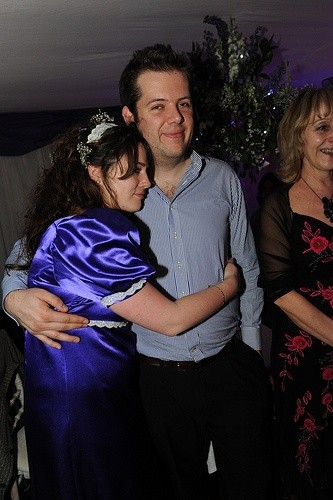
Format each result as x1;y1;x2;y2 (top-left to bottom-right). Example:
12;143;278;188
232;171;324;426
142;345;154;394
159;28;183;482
136;338;236;370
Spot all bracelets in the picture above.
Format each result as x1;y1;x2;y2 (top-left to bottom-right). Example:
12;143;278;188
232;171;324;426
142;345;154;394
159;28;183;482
208;285;226;309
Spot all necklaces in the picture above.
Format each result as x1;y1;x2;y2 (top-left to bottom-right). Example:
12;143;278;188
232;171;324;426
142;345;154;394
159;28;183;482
300;176;333;223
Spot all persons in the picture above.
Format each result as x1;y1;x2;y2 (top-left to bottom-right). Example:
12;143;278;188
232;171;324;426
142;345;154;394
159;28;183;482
23;109;244;500
0;44;267;500
250;85;333;500
